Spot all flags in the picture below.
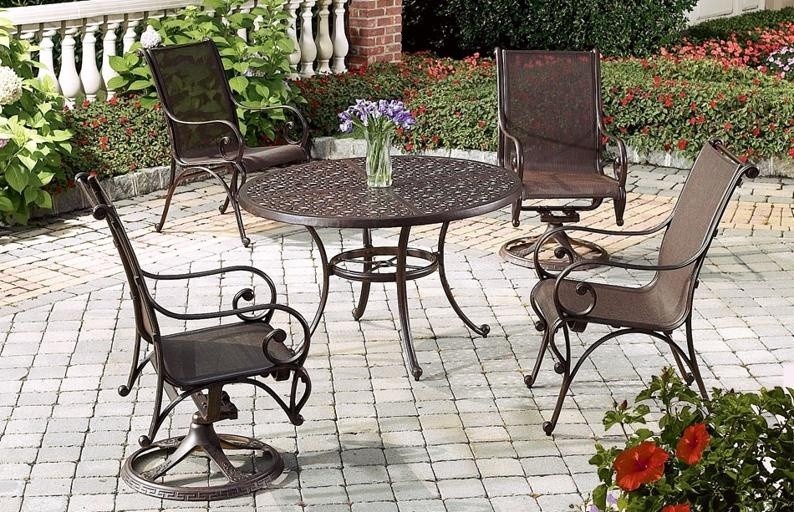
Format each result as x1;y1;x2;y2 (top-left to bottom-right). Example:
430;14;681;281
364;130;393;190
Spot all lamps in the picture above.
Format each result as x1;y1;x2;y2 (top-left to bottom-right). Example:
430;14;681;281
523;136;760;437
61;170;313;505
141;37;313;250
491;45;628;272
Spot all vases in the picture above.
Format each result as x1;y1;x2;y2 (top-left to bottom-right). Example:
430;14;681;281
338;96;416;184
